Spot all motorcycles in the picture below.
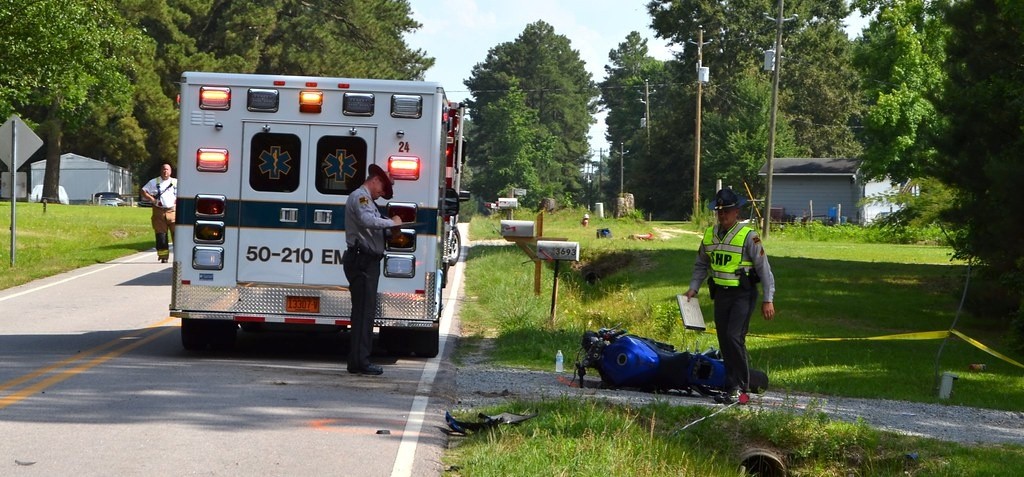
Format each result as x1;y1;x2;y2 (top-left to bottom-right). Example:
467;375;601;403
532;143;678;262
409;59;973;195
568;321;769;399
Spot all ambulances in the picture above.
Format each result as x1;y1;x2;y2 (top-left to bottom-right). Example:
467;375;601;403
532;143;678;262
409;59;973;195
173;70;471;360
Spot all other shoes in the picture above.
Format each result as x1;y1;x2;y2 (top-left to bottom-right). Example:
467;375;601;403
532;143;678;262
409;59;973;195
715;390;746;404
349;365;384;375
162;258;168;263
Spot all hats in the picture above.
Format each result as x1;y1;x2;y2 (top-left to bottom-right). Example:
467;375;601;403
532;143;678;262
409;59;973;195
705;189;748;211
368;163;394;200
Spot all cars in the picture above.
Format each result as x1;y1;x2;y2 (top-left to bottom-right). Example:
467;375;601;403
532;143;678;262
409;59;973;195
89;191;126;206
138;196;153;208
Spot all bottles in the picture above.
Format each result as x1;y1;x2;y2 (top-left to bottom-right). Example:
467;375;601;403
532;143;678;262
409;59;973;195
556;350;564;372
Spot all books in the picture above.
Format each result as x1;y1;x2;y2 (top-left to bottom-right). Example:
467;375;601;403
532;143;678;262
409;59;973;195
677;294;706;331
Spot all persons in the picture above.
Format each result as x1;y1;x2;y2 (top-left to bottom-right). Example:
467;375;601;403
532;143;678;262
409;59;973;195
341;164;401;375
142;164;178;263
684;188;774;402
